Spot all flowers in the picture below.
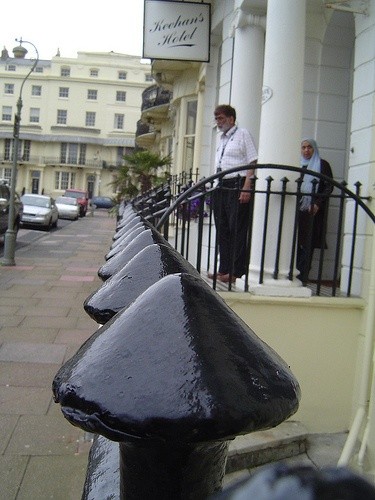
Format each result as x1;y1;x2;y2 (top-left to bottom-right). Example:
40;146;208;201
176;190;214;222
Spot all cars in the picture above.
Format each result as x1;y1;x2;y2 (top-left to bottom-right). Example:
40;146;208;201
0;183;24;248
19;194;59;231
55;196;80;220
89;196;116;209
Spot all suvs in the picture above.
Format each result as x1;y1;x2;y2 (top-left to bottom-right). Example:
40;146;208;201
62;189;88;216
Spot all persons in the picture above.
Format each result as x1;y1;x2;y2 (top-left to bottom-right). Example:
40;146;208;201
297;136;333;287
207;105;259;282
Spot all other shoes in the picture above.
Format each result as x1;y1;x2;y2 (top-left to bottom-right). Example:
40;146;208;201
207;272;235;283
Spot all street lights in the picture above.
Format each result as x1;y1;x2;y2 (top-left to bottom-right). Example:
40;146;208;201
0;37;39;267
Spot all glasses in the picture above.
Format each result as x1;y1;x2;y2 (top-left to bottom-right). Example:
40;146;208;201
215;116;228;120
